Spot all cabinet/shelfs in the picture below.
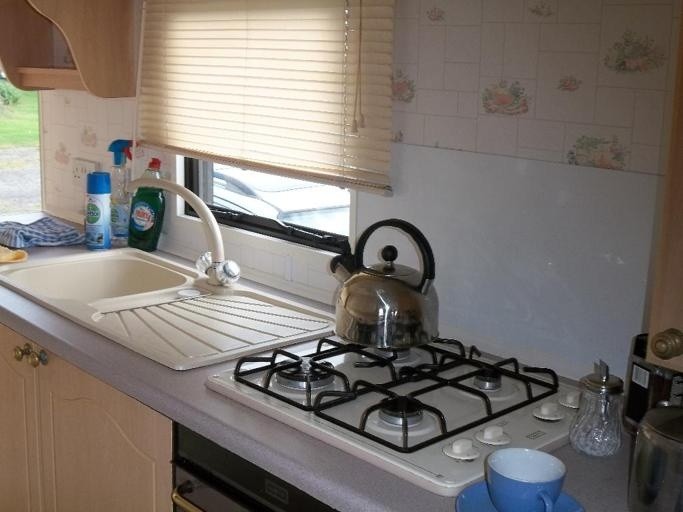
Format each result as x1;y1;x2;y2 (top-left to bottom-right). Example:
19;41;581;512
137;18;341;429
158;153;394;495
0;323;173;512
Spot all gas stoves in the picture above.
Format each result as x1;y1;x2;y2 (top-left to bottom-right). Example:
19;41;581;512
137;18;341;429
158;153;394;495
204;336;584;498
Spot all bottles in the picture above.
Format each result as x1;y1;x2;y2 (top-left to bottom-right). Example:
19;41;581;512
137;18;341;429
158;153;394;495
622;332;673;438
84;171;112;252
569;358;625;458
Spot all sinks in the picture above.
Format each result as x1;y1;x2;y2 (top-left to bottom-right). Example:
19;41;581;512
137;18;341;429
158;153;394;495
0;246;210;301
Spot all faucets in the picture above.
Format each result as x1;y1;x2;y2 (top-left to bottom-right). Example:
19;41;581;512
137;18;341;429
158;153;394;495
126;178;225;285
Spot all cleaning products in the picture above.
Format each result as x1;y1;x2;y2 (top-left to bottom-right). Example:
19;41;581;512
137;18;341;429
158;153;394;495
107;138;140;245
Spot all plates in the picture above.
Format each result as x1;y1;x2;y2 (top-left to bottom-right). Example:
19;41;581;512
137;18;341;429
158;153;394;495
455;480;586;512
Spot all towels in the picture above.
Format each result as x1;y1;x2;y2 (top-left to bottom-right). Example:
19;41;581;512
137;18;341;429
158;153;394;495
0;245;27;263
0;217;86;250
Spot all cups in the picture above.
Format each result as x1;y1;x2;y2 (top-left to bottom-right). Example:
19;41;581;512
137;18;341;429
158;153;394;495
486;446;568;511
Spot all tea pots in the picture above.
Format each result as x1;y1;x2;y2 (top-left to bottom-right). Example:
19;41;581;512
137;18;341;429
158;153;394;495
328;216;440;349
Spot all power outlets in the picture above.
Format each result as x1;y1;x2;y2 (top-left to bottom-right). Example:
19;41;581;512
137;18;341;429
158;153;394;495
63;158;98;188
